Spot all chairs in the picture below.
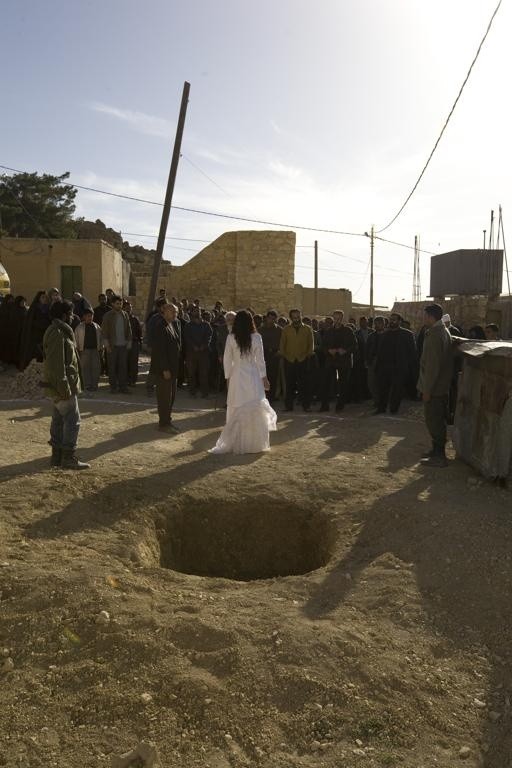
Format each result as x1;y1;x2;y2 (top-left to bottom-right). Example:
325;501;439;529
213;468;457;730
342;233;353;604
62;456;90;469
159;424;180;434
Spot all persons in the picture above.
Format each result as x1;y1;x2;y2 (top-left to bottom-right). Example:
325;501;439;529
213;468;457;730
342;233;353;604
146;301;185;436
441;314;500;340
252;309;427;415
38;299;93;472
414;302;456;470
175;298;237;399
2;287;61;372
206;308;280;456
70;289;143;394
148;289;168;317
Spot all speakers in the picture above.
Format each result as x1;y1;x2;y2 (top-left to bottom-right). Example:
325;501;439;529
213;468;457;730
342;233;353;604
419;442;448;466
